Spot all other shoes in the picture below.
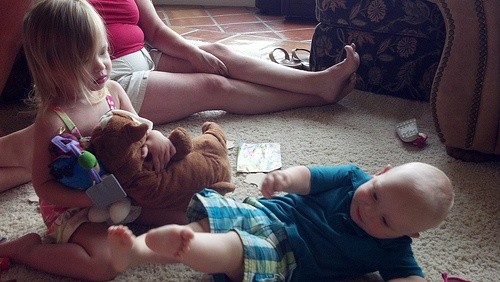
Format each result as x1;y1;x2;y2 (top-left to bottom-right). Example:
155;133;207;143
395;118;419;142
410;133;428;146
441;271;472;282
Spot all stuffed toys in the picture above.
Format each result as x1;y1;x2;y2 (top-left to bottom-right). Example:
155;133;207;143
50;110;236;222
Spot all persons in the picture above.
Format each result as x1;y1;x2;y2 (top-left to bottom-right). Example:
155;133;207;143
108;161;454;282
0;0;177;282
1;1;360;123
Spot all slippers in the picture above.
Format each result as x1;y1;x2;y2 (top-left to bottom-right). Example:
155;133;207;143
265;48;302;67
290;48;311;71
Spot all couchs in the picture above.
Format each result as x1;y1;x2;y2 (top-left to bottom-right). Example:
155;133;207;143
255;0;500;164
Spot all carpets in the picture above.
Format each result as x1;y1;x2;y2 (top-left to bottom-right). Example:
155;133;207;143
0;89;500;282
